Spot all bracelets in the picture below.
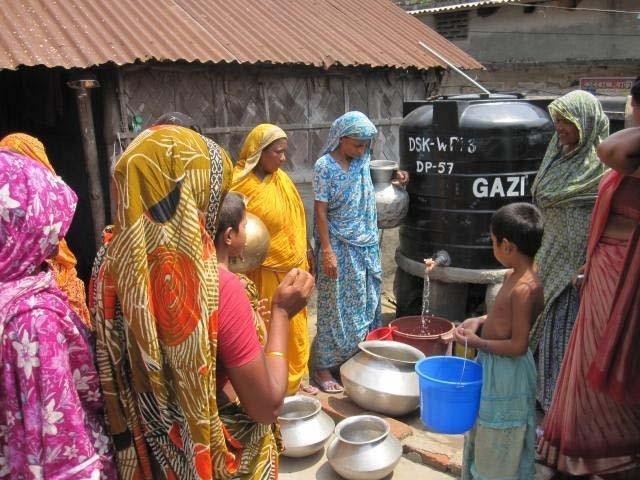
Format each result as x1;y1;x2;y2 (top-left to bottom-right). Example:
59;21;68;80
480;339;490;352
264;352;289;357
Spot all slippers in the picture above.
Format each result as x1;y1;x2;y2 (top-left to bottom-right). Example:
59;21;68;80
302;370;343;394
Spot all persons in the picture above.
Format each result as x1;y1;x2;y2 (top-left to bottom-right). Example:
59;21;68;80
527;88;613;462
89;125;315;480
536;77;639;479
229;123;310;397
451;204;545;480
152;112;202;135
2;133;91;328
214;192;271;352
0;150;120;480
312;109;410;395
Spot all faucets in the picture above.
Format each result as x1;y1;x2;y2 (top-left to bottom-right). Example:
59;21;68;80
422;249;451;273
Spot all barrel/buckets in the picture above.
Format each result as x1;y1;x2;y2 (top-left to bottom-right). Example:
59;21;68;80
388;315;456;358
415;335;483;435
392;92;555;271
365;327;393;341
